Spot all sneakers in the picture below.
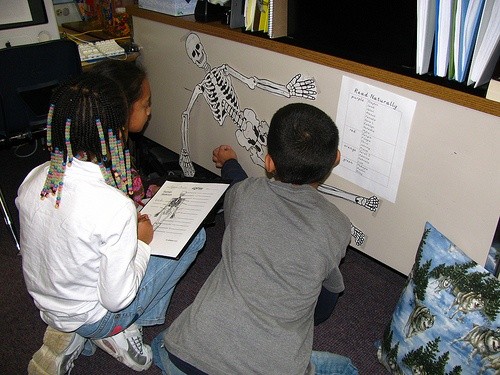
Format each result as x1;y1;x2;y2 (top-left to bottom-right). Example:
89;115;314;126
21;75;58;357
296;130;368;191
92;328;155;372
27;326;85;375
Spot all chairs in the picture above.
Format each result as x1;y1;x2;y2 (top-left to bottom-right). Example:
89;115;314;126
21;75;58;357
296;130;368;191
0;39;83;251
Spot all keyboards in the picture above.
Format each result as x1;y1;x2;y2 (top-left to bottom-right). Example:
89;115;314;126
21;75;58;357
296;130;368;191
78;39;125;62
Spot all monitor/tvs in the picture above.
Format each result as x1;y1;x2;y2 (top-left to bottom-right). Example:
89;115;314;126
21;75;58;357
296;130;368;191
0;0;60;49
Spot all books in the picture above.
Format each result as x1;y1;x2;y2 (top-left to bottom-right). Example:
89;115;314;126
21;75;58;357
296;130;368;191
268;0;287;38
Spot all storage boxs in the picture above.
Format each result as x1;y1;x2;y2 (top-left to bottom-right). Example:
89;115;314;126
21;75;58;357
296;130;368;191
138;0;198;17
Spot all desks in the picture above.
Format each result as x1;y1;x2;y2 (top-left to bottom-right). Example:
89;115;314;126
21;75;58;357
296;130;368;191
58;25;141;72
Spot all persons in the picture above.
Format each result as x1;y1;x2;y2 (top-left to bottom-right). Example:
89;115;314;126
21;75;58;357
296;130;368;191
151;102;359;375
14;60;206;375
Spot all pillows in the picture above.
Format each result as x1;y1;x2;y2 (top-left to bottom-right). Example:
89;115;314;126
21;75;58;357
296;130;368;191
373;222;500;375
